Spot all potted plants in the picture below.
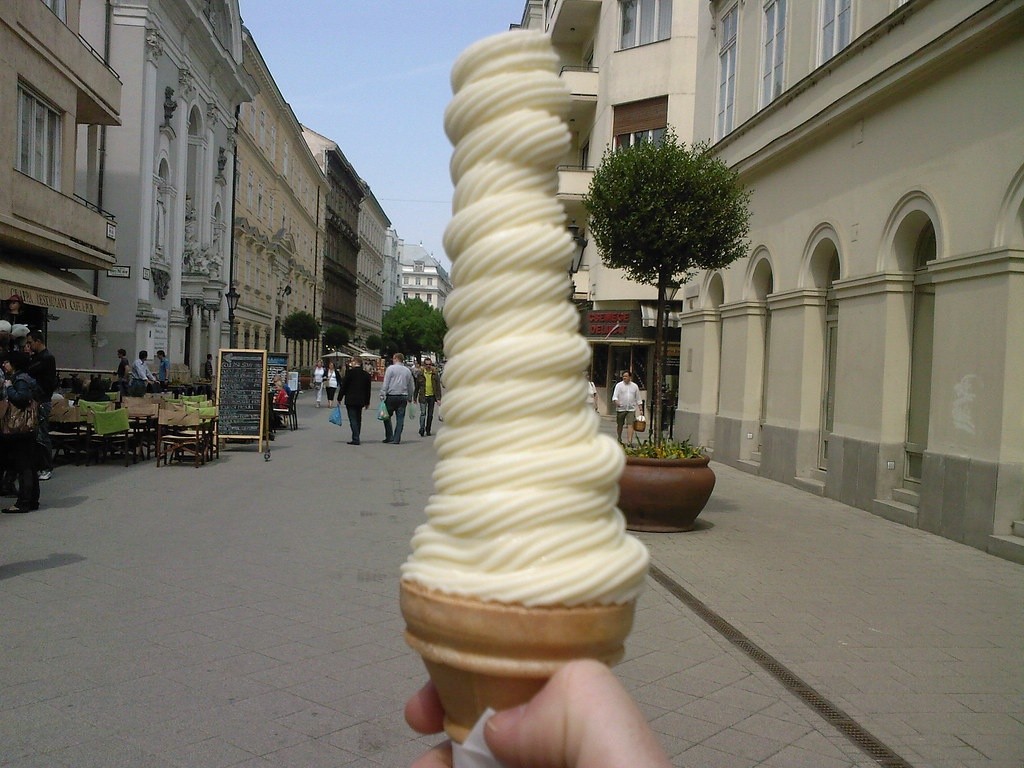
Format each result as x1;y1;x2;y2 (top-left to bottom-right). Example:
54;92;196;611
580;122;757;533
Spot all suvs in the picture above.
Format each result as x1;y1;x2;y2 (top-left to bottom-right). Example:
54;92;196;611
404;360;413;366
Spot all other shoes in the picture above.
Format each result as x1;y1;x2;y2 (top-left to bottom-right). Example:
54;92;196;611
418;428;431;437
2;505;29;514
347;440;360;445
383;437;398;444
39;470;51;480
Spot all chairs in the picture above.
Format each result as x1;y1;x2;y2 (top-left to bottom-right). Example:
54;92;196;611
48;390;300;469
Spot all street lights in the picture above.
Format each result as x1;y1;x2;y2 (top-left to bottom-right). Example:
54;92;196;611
225;284;241;348
357;335;362;348
662;277;681;429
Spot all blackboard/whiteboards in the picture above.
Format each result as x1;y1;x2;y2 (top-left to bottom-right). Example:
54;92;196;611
267;353;289;390
216;349;270;439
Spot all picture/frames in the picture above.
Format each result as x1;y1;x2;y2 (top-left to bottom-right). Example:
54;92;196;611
107;265;131;278
106;222;116;240
142;266;150;280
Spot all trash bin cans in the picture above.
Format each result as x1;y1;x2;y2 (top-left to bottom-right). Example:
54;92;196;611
652;396;667;431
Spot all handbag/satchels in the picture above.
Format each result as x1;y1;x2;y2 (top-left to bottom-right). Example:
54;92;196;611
376;397;389;420
408;402;415;420
0;378;39;435
633;415;646;433
329;403;342;427
436;402;443;422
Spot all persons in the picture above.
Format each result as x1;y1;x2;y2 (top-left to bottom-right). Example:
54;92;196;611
116;349;129;395
611;371;643;449
267;353;446;445
0;330;57;514
5;294;26;325
206;354;214;381
403;656;682;768
130;350;160;397
156;350;171;393
585;373;599;414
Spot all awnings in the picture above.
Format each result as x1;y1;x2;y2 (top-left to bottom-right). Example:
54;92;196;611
0;255;111;318
165;379;168;381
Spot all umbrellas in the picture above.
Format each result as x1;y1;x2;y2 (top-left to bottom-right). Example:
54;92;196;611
321;352;352;358
356;352;381;359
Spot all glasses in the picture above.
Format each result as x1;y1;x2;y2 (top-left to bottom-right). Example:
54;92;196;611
425;363;431;365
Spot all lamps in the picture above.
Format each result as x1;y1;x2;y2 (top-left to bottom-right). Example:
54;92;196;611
277;285;292;297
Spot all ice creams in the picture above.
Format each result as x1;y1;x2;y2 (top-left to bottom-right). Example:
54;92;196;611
397;27;649;747
